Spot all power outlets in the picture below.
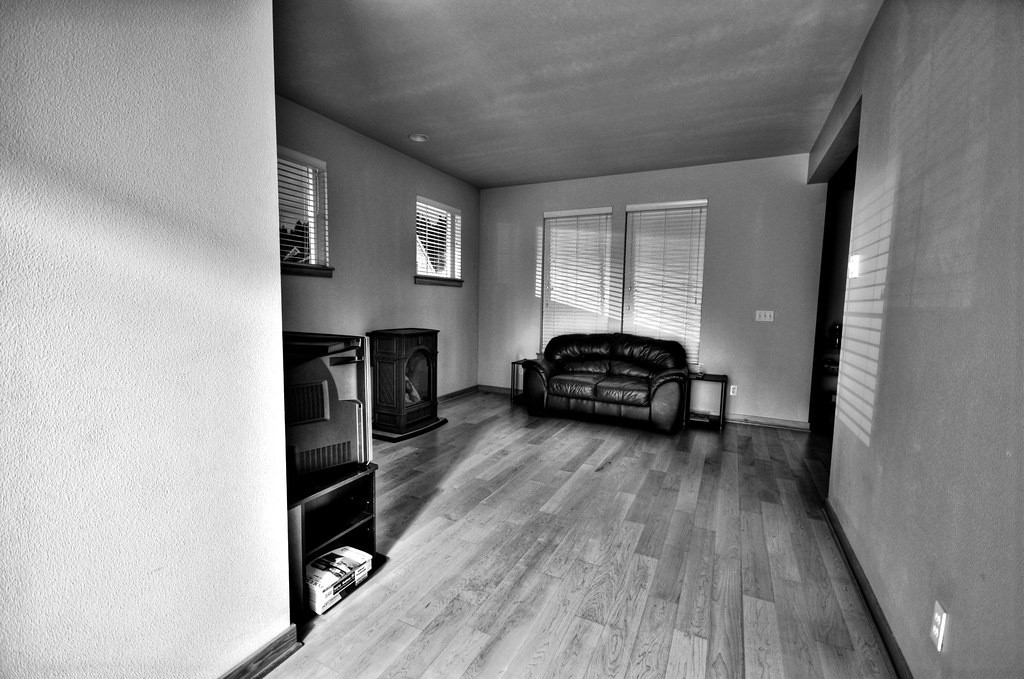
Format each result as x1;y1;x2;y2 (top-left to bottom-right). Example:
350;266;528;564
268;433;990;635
928;599;949;652
730;385;738;396
756;311;774;322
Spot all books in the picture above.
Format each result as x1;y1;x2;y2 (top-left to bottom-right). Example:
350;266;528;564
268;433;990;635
306;545;373;615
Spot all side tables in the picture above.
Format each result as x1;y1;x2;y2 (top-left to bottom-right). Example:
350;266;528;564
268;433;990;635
683;372;728;435
510;358;529;405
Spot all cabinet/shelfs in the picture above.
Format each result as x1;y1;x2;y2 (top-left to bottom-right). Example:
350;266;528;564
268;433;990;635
282;331;378;631
365;328;449;443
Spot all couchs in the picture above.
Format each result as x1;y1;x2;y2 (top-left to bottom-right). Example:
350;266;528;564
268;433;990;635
522;332;688;441
